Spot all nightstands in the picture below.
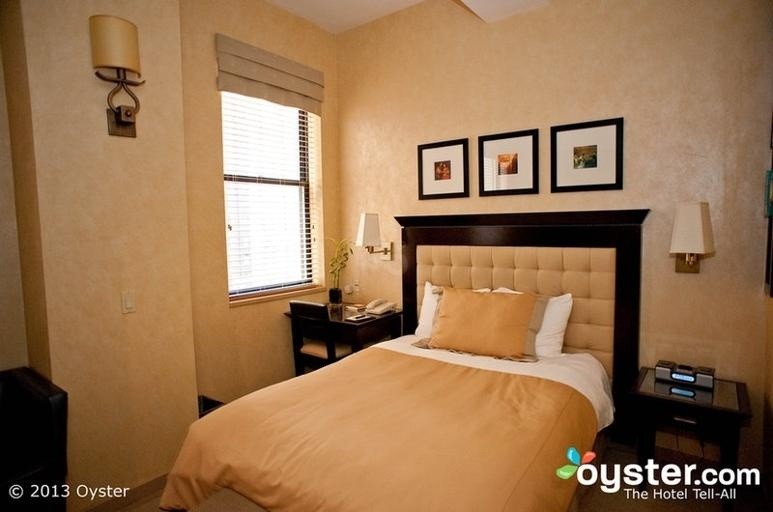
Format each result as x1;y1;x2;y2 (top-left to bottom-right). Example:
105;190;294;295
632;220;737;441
640;365;751;497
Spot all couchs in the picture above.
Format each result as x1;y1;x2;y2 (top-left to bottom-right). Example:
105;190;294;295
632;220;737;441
1;367;67;512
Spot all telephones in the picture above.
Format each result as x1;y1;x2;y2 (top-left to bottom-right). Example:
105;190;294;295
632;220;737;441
364;298;396;315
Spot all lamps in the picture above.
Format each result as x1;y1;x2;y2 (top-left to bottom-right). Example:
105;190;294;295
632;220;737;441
668;201;715;273
88;14;144;138
356;213;392;261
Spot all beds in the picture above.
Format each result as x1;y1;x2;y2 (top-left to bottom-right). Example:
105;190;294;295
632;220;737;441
160;209;651;512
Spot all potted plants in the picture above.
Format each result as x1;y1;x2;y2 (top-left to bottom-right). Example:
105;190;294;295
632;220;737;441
329;237;355;322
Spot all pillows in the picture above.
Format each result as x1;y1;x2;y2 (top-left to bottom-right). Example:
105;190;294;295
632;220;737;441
416;284;490;344
492;287;573;359
428;284;538;360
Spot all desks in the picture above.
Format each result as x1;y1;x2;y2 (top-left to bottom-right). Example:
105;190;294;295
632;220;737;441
285;300;403;352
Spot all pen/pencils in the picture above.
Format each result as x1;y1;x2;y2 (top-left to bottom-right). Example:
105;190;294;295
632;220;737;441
354;314;365;319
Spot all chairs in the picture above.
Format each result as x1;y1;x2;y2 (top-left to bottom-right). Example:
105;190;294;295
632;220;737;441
290;302;353;377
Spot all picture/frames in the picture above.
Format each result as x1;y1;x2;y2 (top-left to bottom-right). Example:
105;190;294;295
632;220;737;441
419;139;469;199
479;129;540;196
551;117;623;193
